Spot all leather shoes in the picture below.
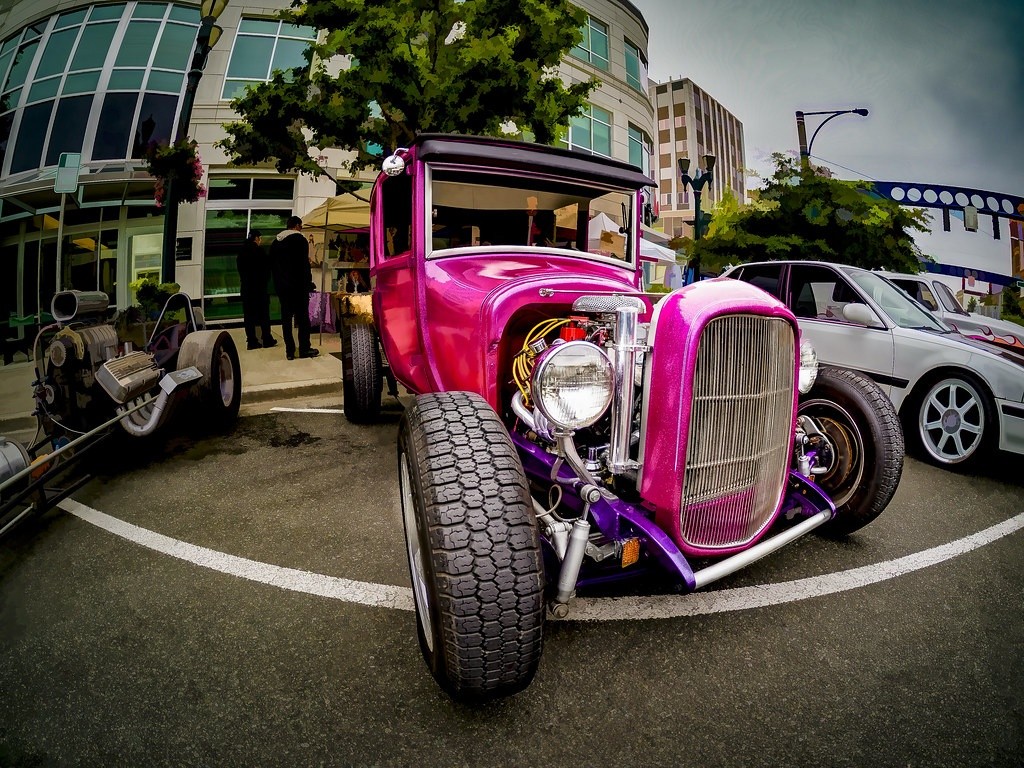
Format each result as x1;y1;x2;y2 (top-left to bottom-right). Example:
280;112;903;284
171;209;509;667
287;352;294;360
299;348;320;358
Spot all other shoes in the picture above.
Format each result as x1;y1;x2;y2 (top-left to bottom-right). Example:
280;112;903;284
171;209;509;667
263;339;277;348
246;342;262;350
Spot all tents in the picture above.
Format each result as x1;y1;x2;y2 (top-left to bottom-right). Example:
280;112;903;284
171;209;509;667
301;179;686;349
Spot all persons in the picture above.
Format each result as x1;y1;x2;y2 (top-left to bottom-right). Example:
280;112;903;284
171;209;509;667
346;271;369;292
270;217;320;361
237;230;278;350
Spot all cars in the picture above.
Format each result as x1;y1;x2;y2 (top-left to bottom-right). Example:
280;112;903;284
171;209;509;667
814;265;1024;352
334;132;904;701
706;259;1024;471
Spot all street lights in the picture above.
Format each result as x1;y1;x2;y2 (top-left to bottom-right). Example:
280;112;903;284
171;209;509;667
793;108;869;179
158;0;231;283
674;154;716;285
